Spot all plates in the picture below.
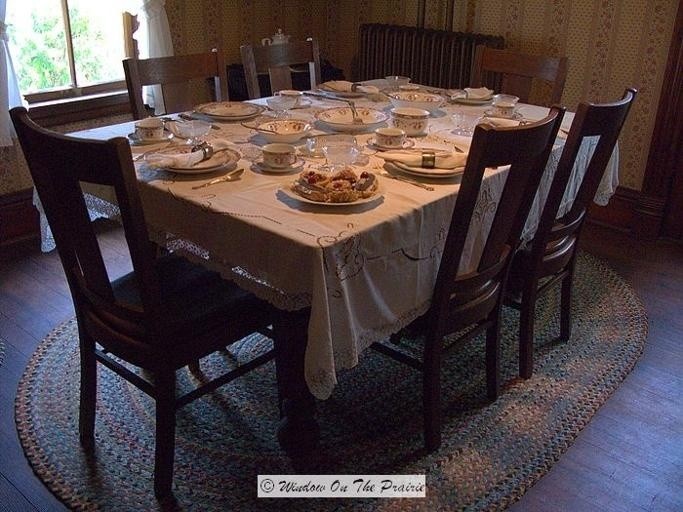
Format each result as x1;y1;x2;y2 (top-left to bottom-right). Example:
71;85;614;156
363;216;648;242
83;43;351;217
126;74;571;179
275;172;383;207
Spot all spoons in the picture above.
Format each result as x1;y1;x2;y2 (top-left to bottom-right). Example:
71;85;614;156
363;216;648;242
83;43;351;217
190;168;246;197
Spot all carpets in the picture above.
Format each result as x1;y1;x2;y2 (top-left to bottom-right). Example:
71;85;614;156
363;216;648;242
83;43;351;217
12;248;647;512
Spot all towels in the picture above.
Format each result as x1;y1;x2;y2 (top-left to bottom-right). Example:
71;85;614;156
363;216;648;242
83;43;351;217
488;116;530;127
147;139;244;169
323;81;378;95
374;143;468;171
451;86;493;100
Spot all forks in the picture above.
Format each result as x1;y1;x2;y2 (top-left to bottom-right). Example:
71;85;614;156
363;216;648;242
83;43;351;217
373;163;435;192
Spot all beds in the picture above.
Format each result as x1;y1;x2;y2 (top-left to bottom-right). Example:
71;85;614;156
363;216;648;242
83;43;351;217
31;78;619;401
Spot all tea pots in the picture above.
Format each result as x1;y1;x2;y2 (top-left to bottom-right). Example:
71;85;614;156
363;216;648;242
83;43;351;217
260;28;291;47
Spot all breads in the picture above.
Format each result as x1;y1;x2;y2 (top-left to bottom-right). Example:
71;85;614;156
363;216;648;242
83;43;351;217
290;166;379;202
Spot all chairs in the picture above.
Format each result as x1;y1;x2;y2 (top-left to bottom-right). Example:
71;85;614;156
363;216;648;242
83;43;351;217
119;43;229;121
470;45;570;107
239;36;321;97
4;104;307;507
510;86;640;375
368;105;565;451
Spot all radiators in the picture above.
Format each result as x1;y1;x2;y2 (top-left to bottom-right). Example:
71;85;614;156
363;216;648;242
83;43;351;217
358;23;504;95
